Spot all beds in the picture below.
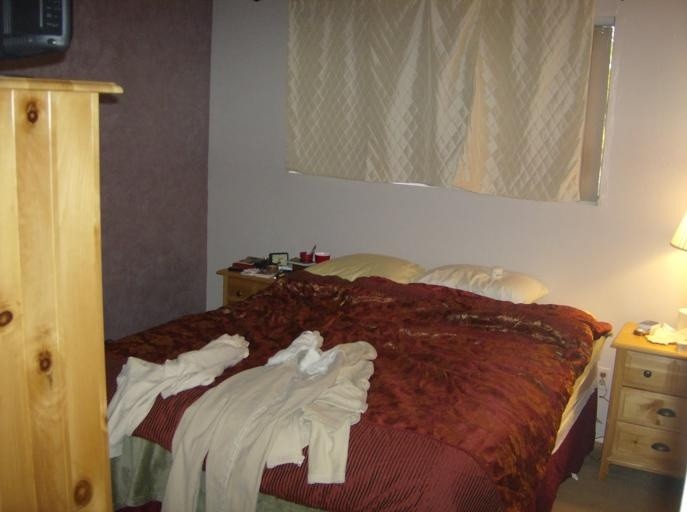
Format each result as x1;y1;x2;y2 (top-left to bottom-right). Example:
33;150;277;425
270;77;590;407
105;270;613;512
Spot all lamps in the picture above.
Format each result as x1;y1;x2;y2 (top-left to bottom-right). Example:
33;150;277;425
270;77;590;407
669;209;687;345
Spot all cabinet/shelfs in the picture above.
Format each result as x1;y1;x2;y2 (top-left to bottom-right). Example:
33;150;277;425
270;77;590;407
598;321;687;480
0;76;123;512
216;268;283;307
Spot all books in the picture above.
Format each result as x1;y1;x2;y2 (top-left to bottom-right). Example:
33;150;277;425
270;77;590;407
240;267;278;280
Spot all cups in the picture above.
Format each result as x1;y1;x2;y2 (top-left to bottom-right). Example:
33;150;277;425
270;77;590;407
316;252;330;264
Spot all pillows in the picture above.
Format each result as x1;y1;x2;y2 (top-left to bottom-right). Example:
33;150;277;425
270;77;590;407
304;253;424;285
416;264;549;304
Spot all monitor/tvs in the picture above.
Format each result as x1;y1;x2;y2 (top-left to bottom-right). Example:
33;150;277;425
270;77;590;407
0;0;72;56
